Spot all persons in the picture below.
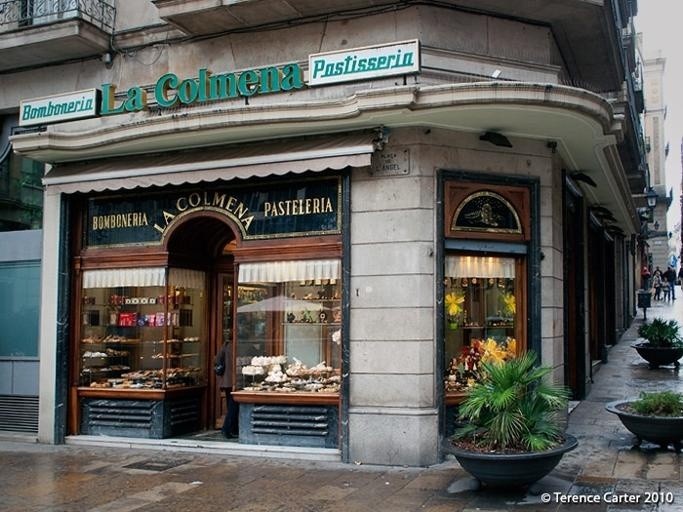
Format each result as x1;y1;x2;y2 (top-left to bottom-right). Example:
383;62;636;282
641;266;651;292
678;267;683;290
652;265;677;301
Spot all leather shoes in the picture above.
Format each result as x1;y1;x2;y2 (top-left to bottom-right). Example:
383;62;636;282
221;429;238;439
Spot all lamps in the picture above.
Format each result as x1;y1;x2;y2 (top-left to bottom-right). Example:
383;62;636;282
640;186;659;238
573;171;597;188
479;131;513;149
591;207;627;240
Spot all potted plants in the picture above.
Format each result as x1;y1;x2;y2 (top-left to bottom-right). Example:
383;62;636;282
605;390;683;454
630;318;683;370
439;350;579;497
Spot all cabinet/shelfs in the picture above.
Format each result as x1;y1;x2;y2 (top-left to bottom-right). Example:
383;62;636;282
77;266;206;388
217;275;271;424
281;278;342;327
447;276;514;338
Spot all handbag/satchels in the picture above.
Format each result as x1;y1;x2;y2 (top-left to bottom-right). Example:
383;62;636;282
214;340;228;377
